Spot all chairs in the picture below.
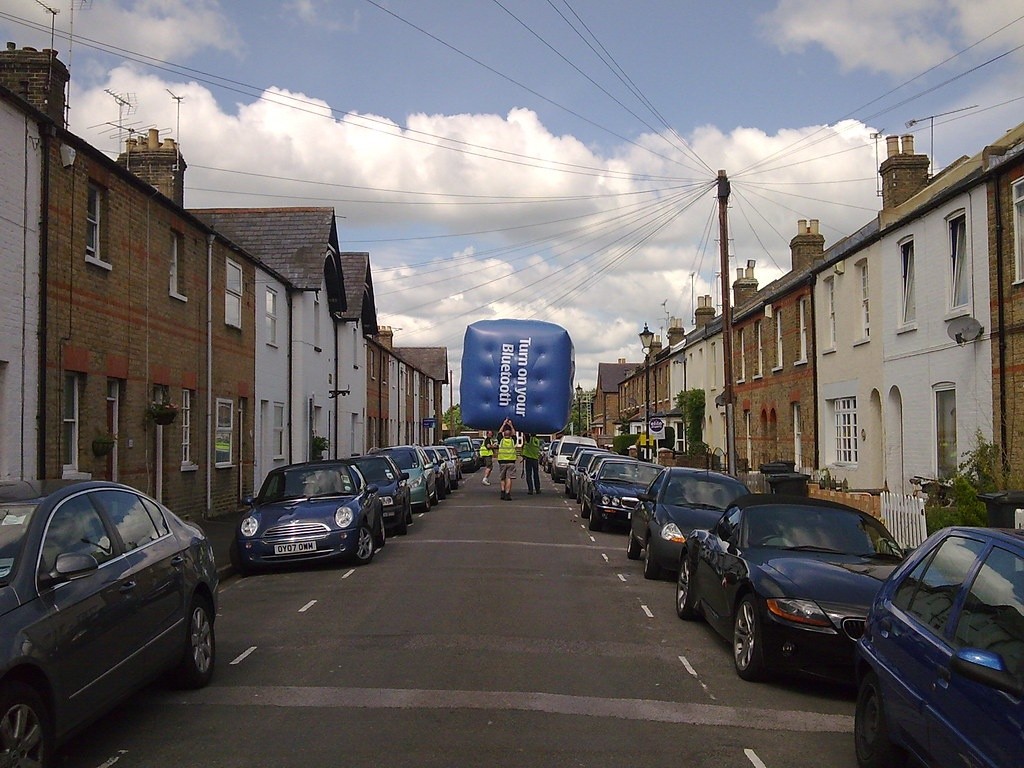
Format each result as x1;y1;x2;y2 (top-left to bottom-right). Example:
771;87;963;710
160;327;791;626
666;483;685;503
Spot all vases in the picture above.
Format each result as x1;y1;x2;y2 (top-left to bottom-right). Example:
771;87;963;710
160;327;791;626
152;414;177;426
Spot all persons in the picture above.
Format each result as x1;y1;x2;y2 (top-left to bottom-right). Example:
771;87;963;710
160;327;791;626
607;445;611;454
479;430;497;486
497;418;517;501
521;432;542;494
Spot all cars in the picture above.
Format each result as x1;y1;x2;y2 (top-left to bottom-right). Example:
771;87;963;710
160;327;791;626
564;446;667;532
627;466;753;580
538;439;560;473
230;454;413;576
458;439;486;468
421;445;462;499
853;526;1024;768
676;491;909;690
1;476;219;768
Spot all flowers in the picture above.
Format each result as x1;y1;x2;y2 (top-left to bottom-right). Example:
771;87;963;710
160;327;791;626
148;394;179;417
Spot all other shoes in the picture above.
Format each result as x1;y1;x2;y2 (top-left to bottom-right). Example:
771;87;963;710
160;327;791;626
536;490;541;494
482;480;490;486
501;490;505;500
528;492;533;495
505;493;512;501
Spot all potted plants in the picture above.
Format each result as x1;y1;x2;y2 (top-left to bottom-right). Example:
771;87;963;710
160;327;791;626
90;425;116;457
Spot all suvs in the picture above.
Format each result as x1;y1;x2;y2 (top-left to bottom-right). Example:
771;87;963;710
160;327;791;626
365;442;439;512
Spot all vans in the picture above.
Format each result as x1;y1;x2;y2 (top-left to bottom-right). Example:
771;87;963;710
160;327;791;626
444;436;478;474
550;436;599;483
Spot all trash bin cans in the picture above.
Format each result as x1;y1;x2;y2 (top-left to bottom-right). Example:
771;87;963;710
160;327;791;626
767;472;811;497
976;489;1024;529
760;461;795;474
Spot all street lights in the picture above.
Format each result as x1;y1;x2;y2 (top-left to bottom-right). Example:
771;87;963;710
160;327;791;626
637;321;655;463
575;382;583;435
